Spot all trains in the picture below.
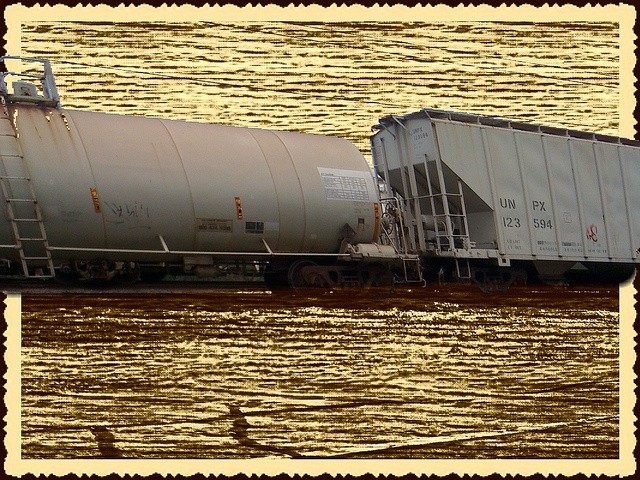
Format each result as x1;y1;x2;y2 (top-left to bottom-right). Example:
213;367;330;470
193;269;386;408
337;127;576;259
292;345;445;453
0;55;640;285
